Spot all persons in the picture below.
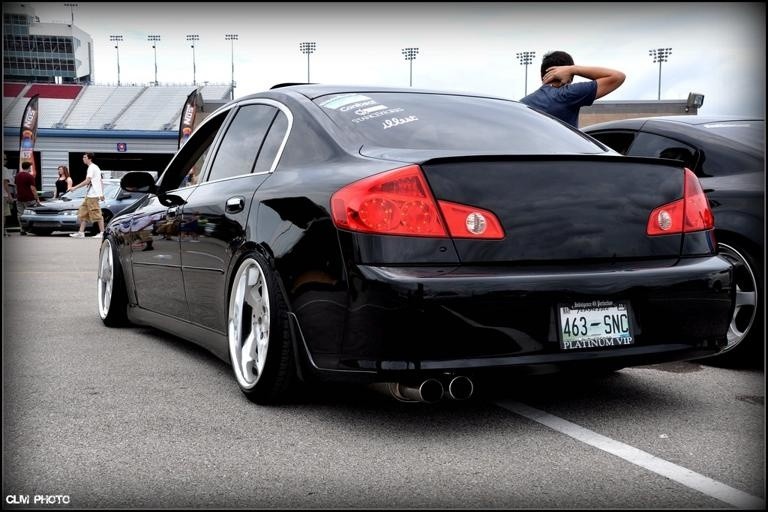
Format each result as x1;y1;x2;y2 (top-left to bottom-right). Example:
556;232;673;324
3;154;14;235
519;49;626;129
15;162;40;235
54;166;73;198
68;152;105;239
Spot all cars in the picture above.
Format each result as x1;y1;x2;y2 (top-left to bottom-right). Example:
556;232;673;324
19;171;158;235
97;83;765;404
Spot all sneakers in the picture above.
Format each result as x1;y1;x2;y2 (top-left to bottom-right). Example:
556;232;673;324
92;232;104;239
69;231;85;239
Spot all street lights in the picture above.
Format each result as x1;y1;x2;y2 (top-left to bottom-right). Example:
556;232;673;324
516;51;535;96
649;48;672;101
300;42;316;83
147;34;161;85
186;34;200;85
402;48;419;87
64;3;78;24
225;34;238;99
110;34;124;85
